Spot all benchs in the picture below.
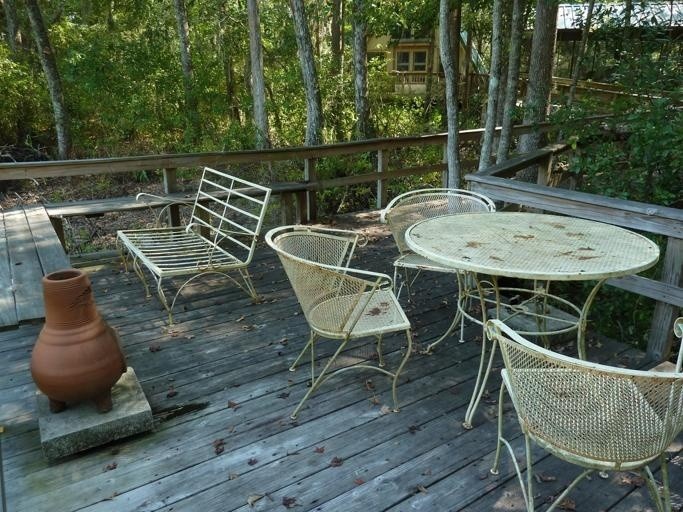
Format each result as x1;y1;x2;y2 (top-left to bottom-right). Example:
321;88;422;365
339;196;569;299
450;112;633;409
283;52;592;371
114;165;272;333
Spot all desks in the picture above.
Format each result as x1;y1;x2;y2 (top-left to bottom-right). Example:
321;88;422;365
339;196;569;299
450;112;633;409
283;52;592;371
404;211;661;431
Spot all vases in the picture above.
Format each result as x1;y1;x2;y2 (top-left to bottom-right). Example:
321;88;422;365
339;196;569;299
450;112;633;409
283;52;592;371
28;269;127;414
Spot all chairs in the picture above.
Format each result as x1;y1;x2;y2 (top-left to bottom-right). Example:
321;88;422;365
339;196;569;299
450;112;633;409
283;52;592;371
263;222;413;422
482;315;683;510
381;186;496;356
